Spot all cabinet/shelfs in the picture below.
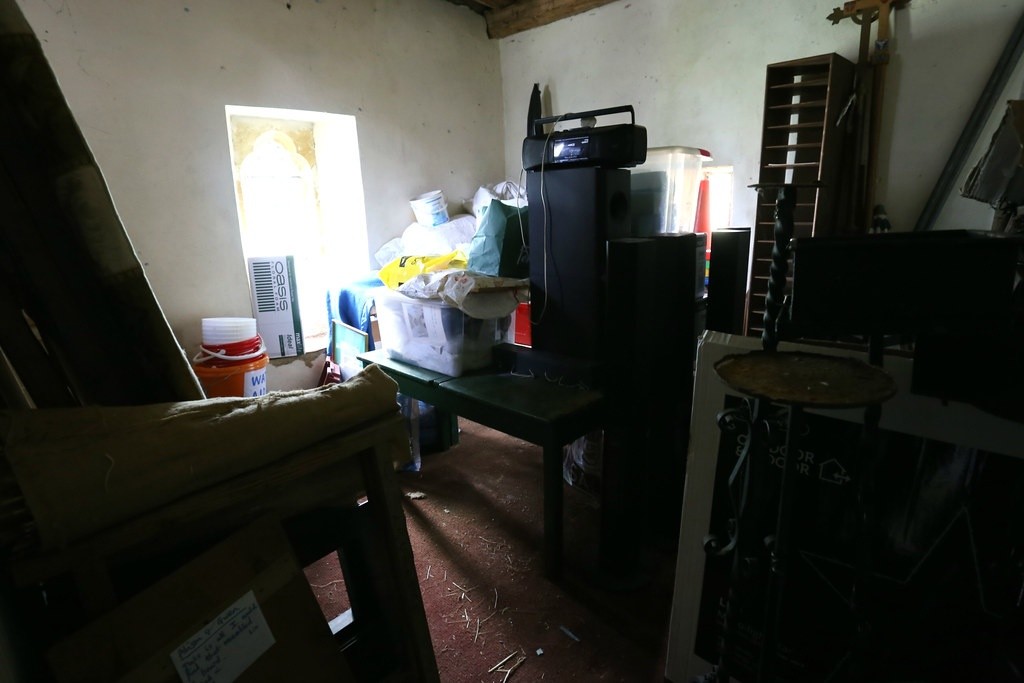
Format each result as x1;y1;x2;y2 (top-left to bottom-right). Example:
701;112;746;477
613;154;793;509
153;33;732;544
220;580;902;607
741;52;856;337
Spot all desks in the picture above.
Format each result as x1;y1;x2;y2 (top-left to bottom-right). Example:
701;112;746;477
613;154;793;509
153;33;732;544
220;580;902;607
359;345;634;563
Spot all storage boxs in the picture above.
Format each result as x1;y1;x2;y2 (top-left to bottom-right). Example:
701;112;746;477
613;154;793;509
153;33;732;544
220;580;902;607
377;289;497;378
46;512;356;683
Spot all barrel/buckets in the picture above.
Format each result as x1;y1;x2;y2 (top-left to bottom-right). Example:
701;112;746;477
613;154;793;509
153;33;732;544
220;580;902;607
192;317;270;399
409;190;451;228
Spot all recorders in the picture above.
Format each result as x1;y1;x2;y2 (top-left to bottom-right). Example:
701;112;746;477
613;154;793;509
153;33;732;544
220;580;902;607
522;105;648;171
